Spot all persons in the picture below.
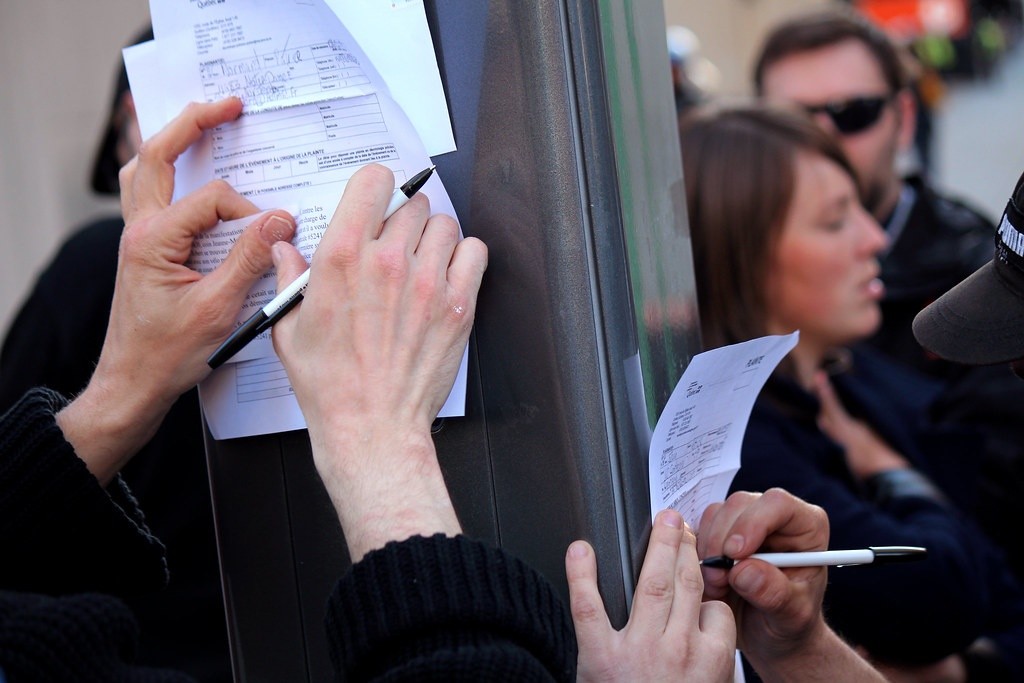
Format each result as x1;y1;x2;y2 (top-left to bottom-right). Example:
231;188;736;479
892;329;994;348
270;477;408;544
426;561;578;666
0;98;569;683
0;28;156;415
565;10;1024;683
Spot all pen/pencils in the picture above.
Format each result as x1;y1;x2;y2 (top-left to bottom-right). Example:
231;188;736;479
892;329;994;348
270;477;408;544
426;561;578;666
699;546;928;569
205;163;439;371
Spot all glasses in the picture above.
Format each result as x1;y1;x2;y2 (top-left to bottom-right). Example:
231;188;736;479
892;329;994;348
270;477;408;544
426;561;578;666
803;96;900;133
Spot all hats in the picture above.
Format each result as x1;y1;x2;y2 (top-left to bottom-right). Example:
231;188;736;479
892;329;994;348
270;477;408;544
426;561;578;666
911;171;1024;363
